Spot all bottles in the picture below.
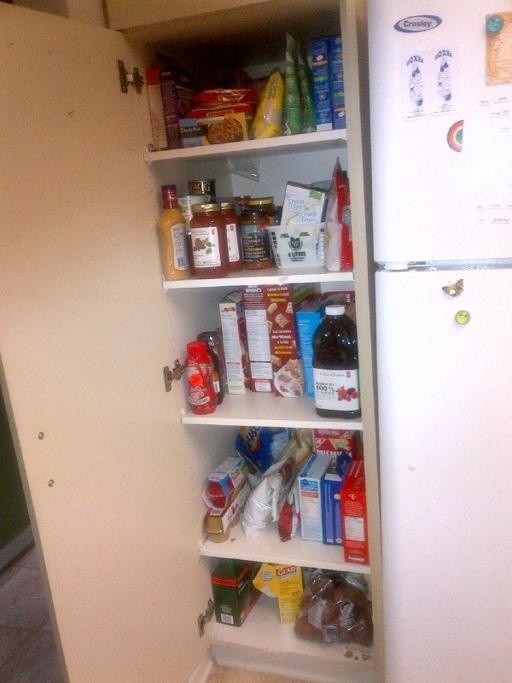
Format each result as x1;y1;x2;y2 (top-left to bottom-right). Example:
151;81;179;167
199;331;225;402
157;182;191;279
185;339;218;415
311;302;360;418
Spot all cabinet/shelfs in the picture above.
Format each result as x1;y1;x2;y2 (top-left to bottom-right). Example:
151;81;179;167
0;0;387;683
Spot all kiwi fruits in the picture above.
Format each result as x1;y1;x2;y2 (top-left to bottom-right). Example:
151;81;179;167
291;576;373;650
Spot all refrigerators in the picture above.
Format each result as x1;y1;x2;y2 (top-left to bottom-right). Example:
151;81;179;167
362;4;508;683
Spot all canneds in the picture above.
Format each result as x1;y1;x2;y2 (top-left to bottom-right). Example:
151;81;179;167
188;178;277;277
